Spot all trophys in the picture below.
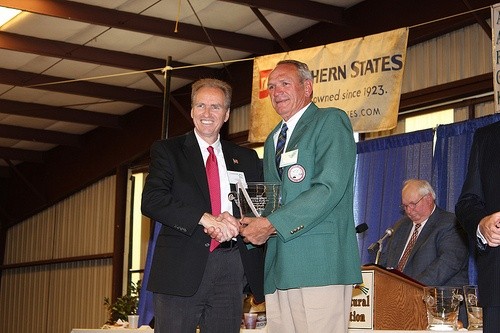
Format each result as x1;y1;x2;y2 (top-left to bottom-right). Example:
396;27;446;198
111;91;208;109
236;182;282;237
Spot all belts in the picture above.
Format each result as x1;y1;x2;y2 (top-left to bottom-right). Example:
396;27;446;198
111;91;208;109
214;240;237;249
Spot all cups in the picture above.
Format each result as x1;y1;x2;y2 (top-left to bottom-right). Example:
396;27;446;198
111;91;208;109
244;313;258;329
128;315;138;329
463;285;483;330
423;286;462;331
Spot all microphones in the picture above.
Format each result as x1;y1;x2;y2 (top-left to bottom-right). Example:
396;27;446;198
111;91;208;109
354;223;369;233
367;228;393;253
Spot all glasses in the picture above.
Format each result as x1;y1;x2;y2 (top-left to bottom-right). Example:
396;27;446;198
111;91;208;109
399;194;428;210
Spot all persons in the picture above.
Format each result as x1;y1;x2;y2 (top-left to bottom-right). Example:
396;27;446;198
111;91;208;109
141;78;266;333
385;178;472;329
203;60;363;333
454;122;500;333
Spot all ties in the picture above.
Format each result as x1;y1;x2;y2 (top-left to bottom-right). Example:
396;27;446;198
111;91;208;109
396;223;422;273
275;123;288;181
207;146;222;250
